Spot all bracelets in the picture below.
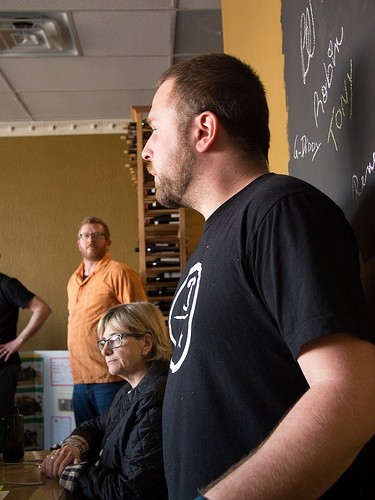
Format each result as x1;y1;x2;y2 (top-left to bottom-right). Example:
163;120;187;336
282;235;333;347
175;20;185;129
60;441;84;455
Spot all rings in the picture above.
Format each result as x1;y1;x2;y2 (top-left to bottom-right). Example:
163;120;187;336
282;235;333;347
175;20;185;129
4;351;6;353
50;455;57;459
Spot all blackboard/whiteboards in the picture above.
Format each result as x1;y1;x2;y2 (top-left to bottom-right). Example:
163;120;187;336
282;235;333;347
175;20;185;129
280;0;375;254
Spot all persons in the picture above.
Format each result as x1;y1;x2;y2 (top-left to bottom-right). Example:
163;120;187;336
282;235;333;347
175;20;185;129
66;216;148;424
0;272;52;418
141;53;375;500
40;300;174;500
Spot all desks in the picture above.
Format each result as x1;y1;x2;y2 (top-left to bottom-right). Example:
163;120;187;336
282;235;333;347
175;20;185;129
0;449;75;500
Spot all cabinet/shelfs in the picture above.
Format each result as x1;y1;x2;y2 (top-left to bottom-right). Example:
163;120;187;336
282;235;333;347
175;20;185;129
133;103;187;312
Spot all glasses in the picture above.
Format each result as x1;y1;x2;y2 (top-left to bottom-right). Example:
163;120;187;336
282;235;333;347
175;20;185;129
98;332;145;352
80;233;108;240
